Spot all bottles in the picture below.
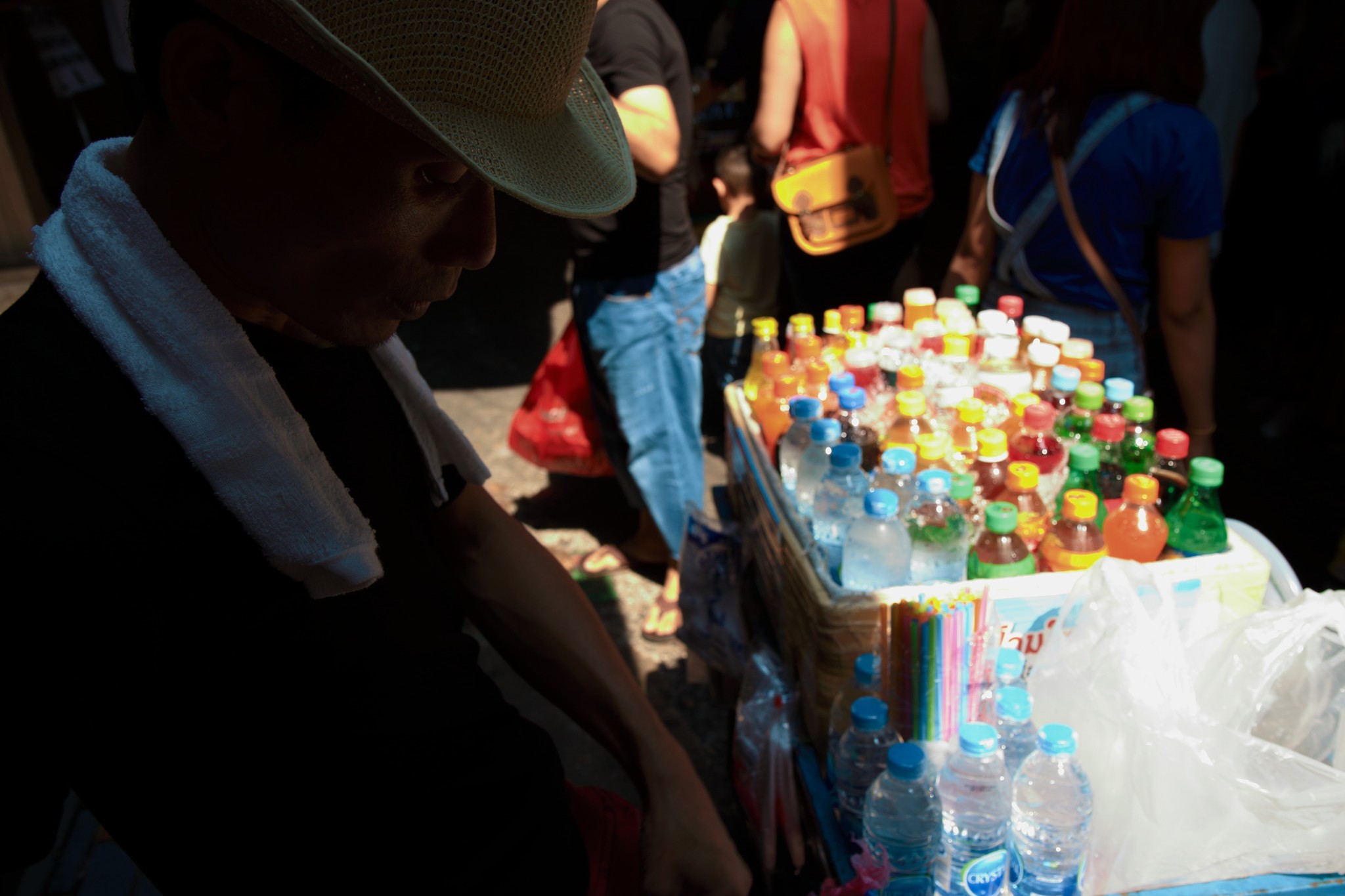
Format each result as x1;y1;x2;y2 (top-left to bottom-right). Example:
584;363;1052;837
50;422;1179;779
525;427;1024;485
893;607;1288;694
827;654;883;784
742;287;1227;591
836;697;904;852
997;647;1024;687
1013;723;1091;896
863;742;941;896
995;687;1041;778
937;721;1014;896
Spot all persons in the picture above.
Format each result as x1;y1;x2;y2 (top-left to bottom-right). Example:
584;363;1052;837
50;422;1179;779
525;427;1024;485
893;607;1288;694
752;0;951;346
695;142;781;431
941;1;1223;470
0;0;754;896
568;2;711;646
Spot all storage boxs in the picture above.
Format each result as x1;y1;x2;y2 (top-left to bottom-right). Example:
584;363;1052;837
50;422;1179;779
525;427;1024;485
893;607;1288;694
721;375;1274;765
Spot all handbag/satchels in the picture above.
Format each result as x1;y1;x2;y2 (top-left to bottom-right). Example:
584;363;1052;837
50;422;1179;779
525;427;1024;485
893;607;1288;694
509;313;616;478
771;142;898;257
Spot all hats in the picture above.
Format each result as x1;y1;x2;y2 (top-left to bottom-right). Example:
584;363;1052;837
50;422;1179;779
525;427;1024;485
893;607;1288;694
187;0;639;221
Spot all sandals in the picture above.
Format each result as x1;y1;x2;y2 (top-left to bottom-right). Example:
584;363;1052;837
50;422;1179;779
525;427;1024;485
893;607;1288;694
643;594;683;641
579;541;652;575
